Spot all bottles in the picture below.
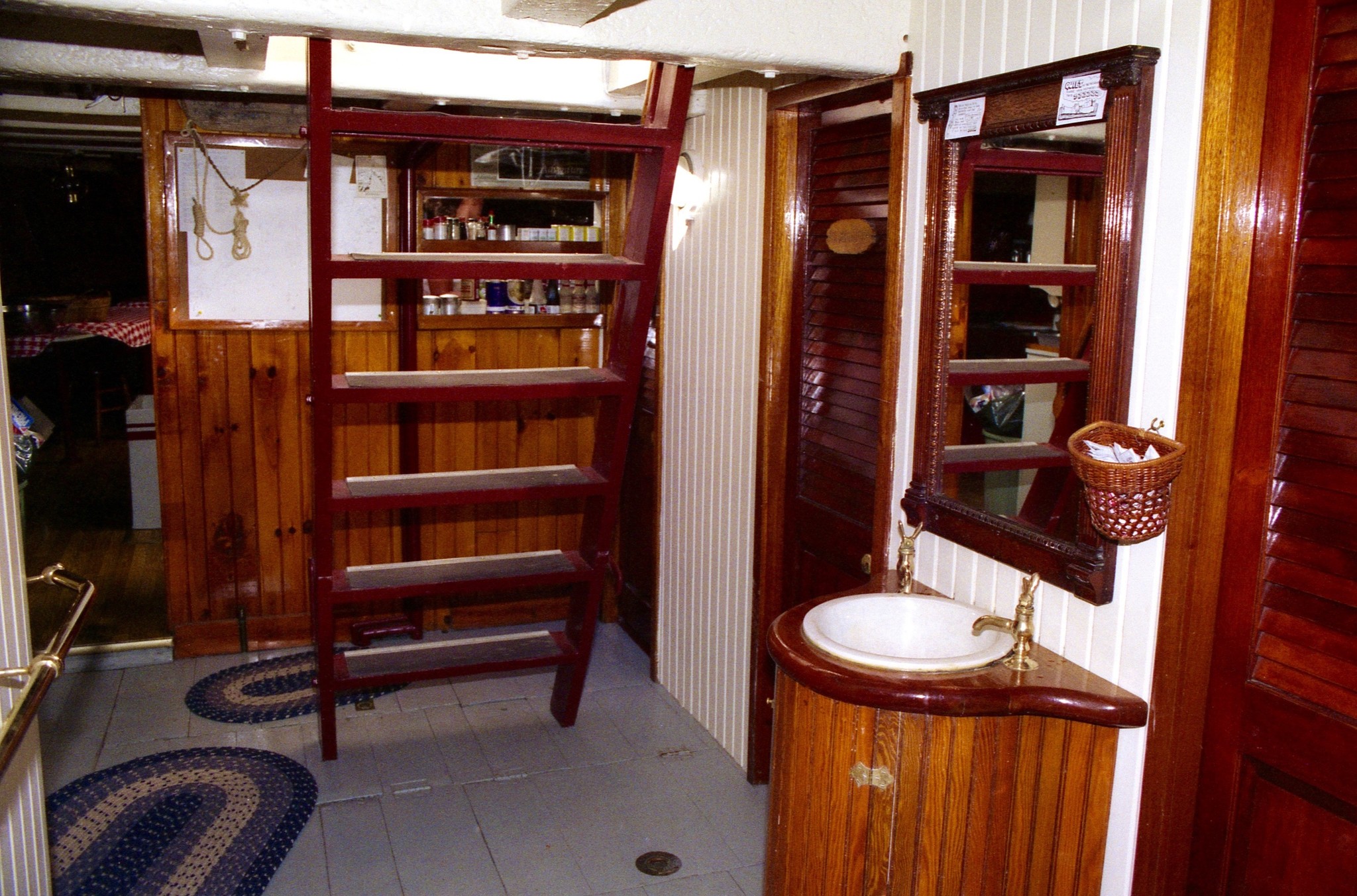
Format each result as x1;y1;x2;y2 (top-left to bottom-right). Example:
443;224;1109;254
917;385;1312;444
423;215;500;240
586;279;599;313
559;277;572;313
546;279;561;305
530;279;547;304
573;281;586;314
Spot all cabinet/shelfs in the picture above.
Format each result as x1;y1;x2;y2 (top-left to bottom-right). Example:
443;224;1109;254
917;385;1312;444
407;185;603;316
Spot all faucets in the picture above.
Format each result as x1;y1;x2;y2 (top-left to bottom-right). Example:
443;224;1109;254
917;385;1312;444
895;520;924;594
971;570;1040;673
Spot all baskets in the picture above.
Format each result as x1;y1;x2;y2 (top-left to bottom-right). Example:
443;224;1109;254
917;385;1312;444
1067;420;1187;544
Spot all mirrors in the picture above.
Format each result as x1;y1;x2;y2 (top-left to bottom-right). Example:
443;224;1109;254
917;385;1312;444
913;43;1162;605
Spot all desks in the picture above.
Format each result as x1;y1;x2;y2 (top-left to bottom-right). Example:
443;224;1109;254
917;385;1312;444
5;302;150;441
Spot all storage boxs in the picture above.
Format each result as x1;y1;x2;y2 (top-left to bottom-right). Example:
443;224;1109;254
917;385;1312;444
125;394;162;529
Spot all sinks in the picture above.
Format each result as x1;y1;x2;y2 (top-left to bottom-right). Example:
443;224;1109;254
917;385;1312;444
802;591;1016;672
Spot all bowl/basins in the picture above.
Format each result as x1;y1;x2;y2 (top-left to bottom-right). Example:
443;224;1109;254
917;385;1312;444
3;300;67;335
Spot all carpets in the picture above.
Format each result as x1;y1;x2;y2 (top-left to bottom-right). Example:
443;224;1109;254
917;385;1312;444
185;647;410;724
45;745;318;896
